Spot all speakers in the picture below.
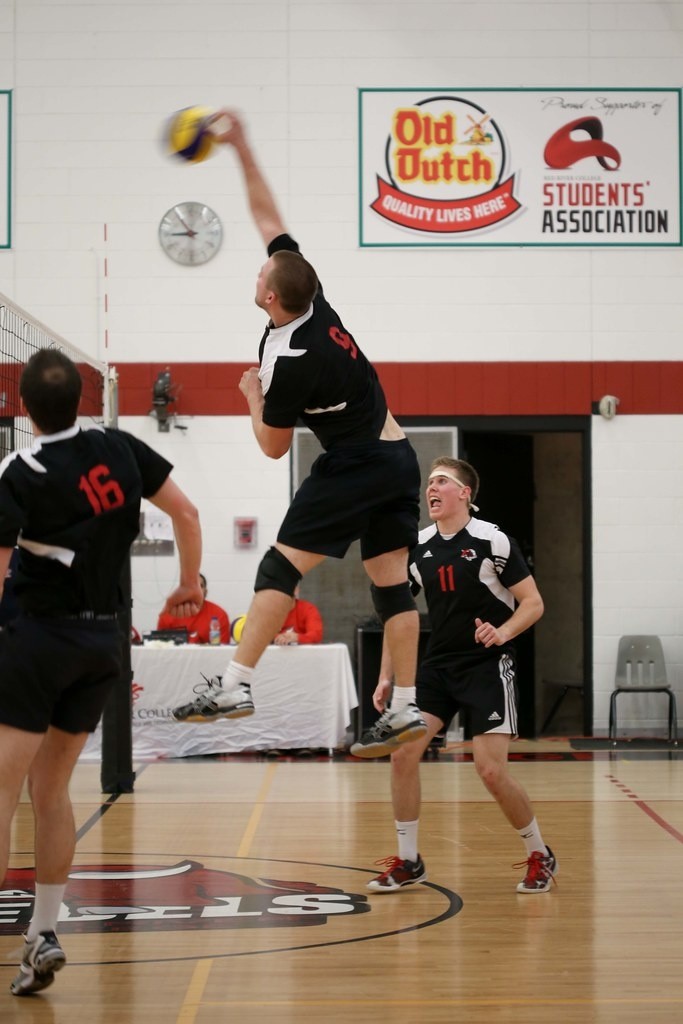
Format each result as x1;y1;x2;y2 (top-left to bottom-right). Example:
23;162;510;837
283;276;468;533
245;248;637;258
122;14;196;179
355;626;446;748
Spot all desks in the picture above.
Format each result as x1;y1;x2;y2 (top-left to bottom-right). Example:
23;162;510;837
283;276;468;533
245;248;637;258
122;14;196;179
76;643;360;758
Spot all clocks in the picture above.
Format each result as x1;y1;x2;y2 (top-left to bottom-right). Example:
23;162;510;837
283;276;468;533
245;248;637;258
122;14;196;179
159;202;223;268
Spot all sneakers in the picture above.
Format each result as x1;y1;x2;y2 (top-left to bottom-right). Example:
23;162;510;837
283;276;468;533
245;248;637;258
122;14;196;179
365;856;428;895
172;672;255;722
348;701;430;759
10;930;67;1006
512;846;557;893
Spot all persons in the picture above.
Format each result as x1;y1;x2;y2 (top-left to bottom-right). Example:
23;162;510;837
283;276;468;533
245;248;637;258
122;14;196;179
0;348;204;995
170;108;429;759
367;455;561;894
156;574;324;646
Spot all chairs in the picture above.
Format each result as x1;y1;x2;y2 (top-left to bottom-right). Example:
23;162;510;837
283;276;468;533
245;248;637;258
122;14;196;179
609;636;679;747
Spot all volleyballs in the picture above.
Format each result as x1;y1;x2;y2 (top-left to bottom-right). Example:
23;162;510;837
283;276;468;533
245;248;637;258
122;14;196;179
231;615;248;644
162;105;219;165
